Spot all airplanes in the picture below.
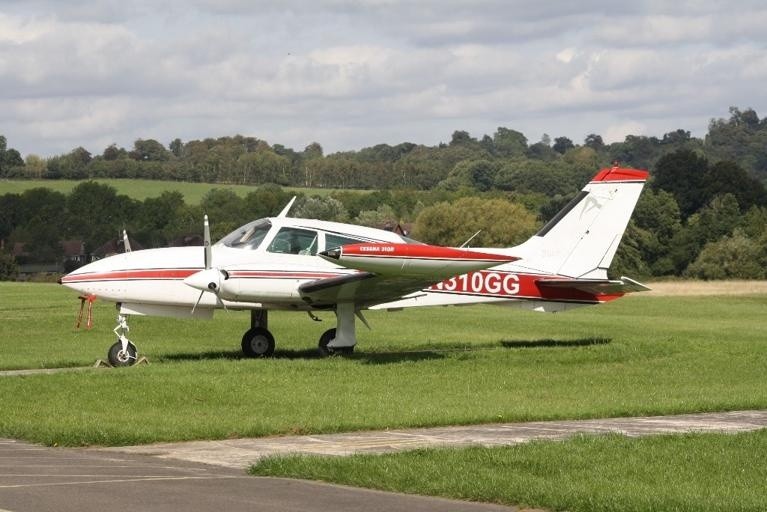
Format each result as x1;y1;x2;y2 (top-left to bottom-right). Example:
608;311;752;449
58;160;651;367
